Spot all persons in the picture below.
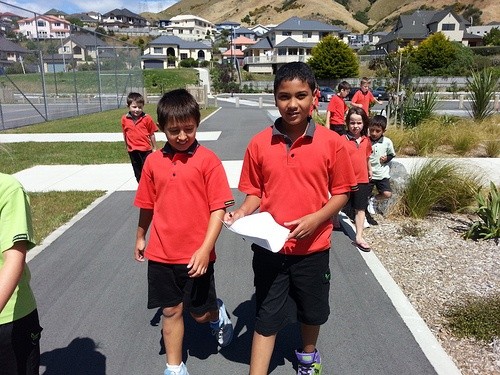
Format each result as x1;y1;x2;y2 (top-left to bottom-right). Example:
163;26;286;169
223;61;359;375
325;77;395;252
0;171;43;375
134;89;236;375
122;92;159;184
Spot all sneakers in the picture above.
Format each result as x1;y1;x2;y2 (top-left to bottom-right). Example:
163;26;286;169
164;363;191;375
364;217;370;228
367;196;376;215
295;347;322;375
210;298;234;348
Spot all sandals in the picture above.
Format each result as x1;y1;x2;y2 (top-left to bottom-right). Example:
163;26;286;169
352;234;371;252
334;219;340;229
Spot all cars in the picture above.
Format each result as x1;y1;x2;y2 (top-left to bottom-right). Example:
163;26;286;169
318;87;337;102
344;87;373;101
371;86;389;100
